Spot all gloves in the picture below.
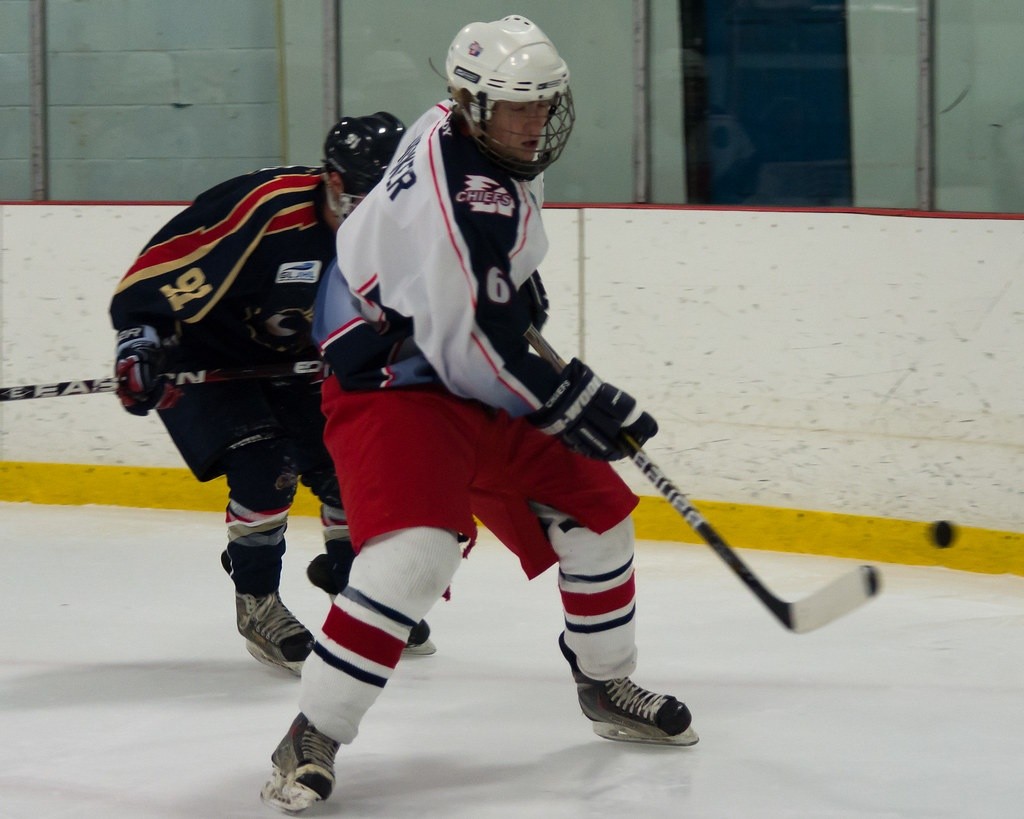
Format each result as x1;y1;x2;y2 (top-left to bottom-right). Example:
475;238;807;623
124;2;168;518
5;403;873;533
525;357;658;461
114;327;183;417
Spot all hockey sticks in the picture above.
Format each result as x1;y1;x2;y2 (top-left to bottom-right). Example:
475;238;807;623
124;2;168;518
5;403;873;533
526;325;884;637
0;357;335;406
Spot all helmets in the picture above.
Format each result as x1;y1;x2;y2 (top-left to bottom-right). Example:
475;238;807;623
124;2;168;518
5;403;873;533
445;13;576;182
324;112;404;223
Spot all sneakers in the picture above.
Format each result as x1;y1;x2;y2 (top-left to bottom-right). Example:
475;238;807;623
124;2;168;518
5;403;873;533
559;631;700;747
307;554;438;656
259;711;343;815
221;549;314;676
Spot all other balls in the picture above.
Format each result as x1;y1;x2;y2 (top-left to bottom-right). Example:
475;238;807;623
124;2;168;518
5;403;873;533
924;519;958;550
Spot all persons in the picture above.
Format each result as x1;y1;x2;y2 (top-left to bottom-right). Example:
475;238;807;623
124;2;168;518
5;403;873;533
260;13;702;814
109;110;437;678
